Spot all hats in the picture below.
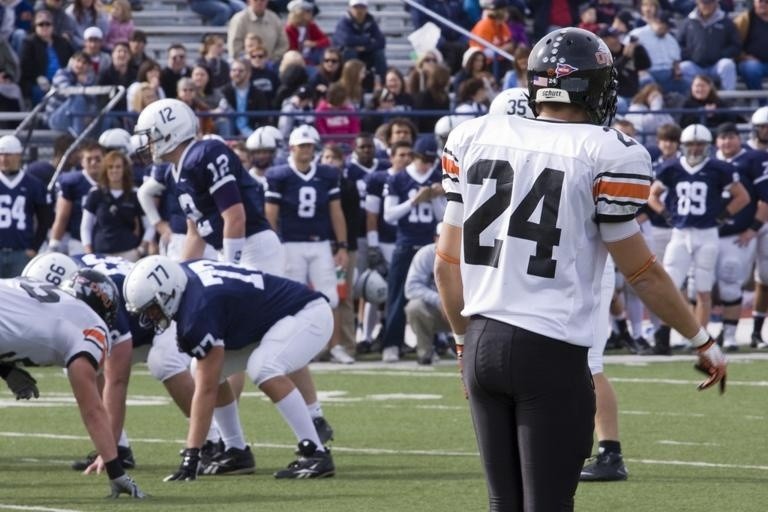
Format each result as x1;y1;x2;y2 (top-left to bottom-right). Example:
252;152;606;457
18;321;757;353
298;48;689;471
83;26;103;41
751;105;767;126
348;0;369;7
413;133;438;159
288;124;320;146
461;46;481;69
0;134;24;155
679;123;713;145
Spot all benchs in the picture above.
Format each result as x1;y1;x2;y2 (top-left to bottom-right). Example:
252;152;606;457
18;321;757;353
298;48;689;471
0;0;422;82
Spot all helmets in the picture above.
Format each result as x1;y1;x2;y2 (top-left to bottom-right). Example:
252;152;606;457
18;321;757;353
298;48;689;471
525;26;620;125
97;127;132;153
134;96;200;166
21;250;121;332
123;253;189;336
245;125;285;152
356;266;389;305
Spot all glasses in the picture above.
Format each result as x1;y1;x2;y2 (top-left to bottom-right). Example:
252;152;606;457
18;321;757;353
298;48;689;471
251;55;264;59
35;21;53;27
324;58;337;64
172;55;185;60
424;56;437;63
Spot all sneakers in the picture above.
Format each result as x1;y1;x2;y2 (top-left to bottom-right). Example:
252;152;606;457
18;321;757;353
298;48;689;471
607;331;672;355
312;416;334;445
201;438;258;476
358;340;372;353
722;330;740;351
274;438;336;480
579;446;628;482
72;443;136;474
749;333;767;349
381;344;400;363
328;344;355;364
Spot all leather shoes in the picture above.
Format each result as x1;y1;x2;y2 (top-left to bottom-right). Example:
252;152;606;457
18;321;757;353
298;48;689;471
415;351;432;365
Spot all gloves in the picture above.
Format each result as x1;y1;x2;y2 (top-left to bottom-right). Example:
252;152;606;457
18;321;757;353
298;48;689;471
103;456;145;500
367;246;385;268
162;447;203;484
689;325;727;396
0;361;39;401
